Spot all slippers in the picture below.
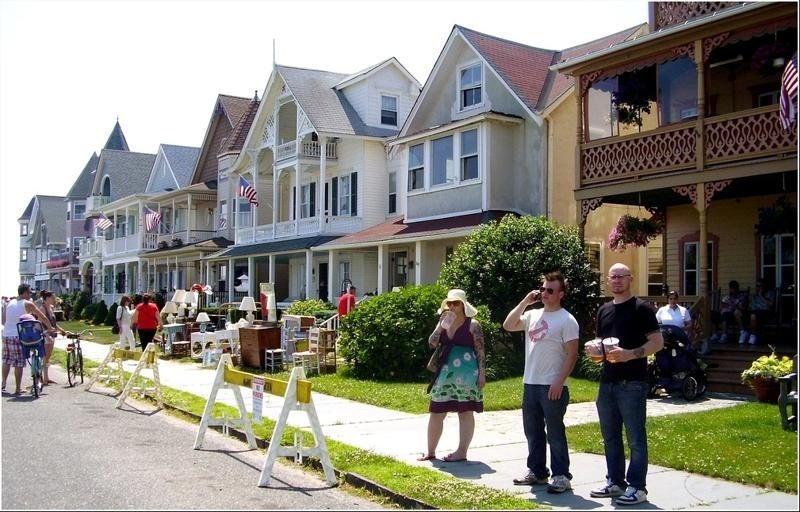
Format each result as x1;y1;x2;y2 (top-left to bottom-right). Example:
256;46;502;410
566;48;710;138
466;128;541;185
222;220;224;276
418;454;437;461
443;453;468;462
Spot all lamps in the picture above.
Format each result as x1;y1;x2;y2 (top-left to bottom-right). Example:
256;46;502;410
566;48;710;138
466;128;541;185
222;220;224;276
239;296;257;324
265;293;276;321
172;289;188;317
185;292;196;318
162;302;178;322
195;311;211;333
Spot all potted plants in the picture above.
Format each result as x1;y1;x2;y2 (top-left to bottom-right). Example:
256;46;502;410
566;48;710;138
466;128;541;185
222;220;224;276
741;346;793;401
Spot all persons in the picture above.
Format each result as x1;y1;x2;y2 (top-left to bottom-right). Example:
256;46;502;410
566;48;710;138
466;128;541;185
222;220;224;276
654;290;693;337
582;262;666;507
415;287;486;463
115;295;136;352
710;280;745;343
1;282;56;394
737;276;774;345
501;270;579;493
338;285;357;320
130;293;164;352
37;289;67;385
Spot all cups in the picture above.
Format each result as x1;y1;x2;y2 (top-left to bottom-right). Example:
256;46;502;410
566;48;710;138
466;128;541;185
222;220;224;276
603;337;620;361
441;311;457;329
585;338;604;362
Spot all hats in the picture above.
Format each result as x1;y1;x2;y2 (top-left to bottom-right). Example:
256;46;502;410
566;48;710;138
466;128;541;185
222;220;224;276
437;289;479;320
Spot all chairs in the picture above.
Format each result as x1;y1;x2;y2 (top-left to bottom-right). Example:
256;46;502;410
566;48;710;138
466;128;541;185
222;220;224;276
704;287;785;333
341;277;353;296
155;316;339;378
776;352;797;430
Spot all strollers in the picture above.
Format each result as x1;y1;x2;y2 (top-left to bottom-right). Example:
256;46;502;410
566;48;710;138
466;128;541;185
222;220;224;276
645;324;708;402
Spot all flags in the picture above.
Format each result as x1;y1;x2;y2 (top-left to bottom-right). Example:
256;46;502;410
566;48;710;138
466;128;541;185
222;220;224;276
239;175;259;207
145;206;160;231
95;213;112;231
776;51;798;132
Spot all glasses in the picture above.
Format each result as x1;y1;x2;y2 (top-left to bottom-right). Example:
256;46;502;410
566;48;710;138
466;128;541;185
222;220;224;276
607;275;630;280
540;286;559;295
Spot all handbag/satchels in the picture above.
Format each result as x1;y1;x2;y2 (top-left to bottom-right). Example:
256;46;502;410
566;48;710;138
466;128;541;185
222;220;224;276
427;343;442;376
111;324;120;335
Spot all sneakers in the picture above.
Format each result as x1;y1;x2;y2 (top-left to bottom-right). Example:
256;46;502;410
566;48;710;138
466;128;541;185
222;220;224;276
711;334;719;341
590;481;625;497
15;390;26;395
748;334;757;345
2;388;10;395
738;331;748;344
719;335;728;343
513;469;549;485
43;380;57;385
547;475;572;494
615;485;647;505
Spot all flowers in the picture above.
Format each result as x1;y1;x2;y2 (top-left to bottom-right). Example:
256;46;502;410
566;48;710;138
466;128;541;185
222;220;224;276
607;205;668;255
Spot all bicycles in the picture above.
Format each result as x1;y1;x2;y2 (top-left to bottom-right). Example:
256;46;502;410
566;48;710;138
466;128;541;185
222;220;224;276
18;315;50;398
53;329;95;387
